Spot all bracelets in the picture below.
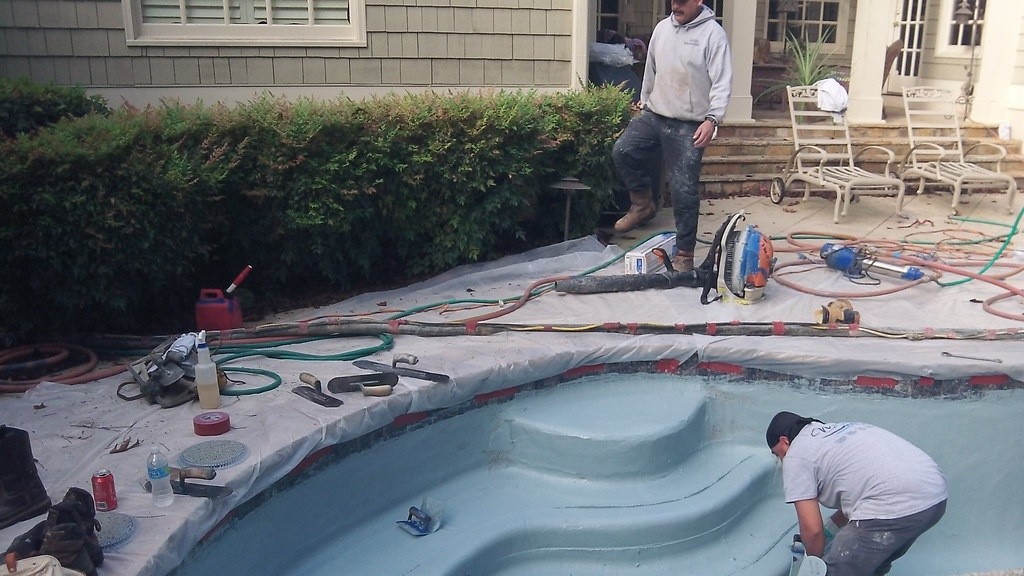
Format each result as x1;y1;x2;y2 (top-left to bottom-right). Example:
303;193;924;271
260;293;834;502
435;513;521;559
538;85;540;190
706;117;716;126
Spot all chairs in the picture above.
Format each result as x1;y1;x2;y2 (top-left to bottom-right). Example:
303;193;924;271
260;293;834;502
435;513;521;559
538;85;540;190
885;85;1017;216
771;84;906;224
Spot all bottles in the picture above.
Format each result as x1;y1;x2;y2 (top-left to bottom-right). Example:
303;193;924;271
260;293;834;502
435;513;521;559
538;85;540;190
195;342;220;409
147;446;174;507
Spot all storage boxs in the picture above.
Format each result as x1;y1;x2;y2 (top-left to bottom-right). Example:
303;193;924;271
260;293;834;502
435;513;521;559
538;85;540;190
625;233;676;274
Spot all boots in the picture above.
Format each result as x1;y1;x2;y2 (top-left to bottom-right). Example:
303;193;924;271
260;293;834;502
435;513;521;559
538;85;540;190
615;188;657;230
661;251;699;286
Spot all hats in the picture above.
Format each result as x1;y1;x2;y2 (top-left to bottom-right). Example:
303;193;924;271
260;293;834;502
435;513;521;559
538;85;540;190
766;411;816;443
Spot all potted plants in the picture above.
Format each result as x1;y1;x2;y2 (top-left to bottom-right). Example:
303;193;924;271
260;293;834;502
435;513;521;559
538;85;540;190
753;24;843;123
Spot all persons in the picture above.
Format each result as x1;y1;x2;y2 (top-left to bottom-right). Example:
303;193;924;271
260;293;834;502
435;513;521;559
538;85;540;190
764;410;951;576
610;0;733;274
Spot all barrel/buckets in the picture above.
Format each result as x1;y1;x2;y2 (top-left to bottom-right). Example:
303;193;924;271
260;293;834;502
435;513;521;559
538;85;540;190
195;288;241;331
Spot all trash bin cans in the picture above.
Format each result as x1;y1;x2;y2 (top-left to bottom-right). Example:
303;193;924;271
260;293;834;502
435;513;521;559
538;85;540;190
589;59;644;105
